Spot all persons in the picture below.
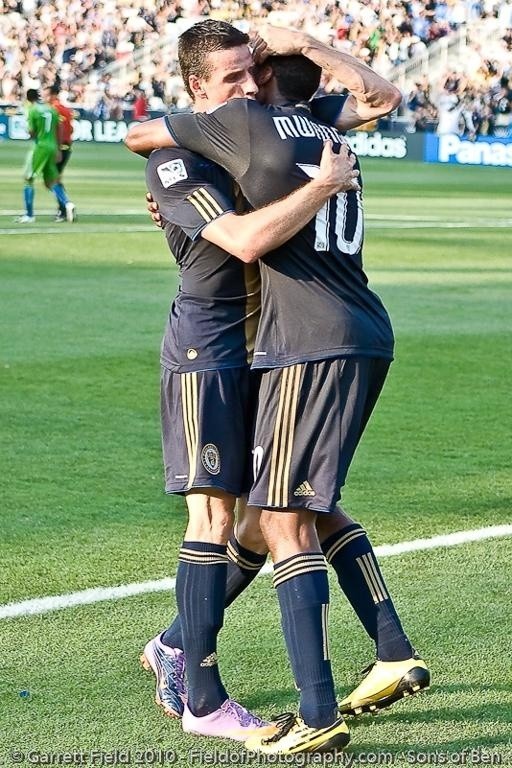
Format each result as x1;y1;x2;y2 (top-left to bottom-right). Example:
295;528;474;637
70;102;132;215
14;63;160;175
43;85;72;223
1;1;511;142
124;51;432;754
137;18;407;748
14;89;78;225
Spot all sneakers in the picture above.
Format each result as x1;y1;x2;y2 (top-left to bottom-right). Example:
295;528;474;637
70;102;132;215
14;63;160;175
53;200;77;225
242;704;351;756
337;645;431;718
140;628;187;720
181;694;281;743
14;214;36;224
